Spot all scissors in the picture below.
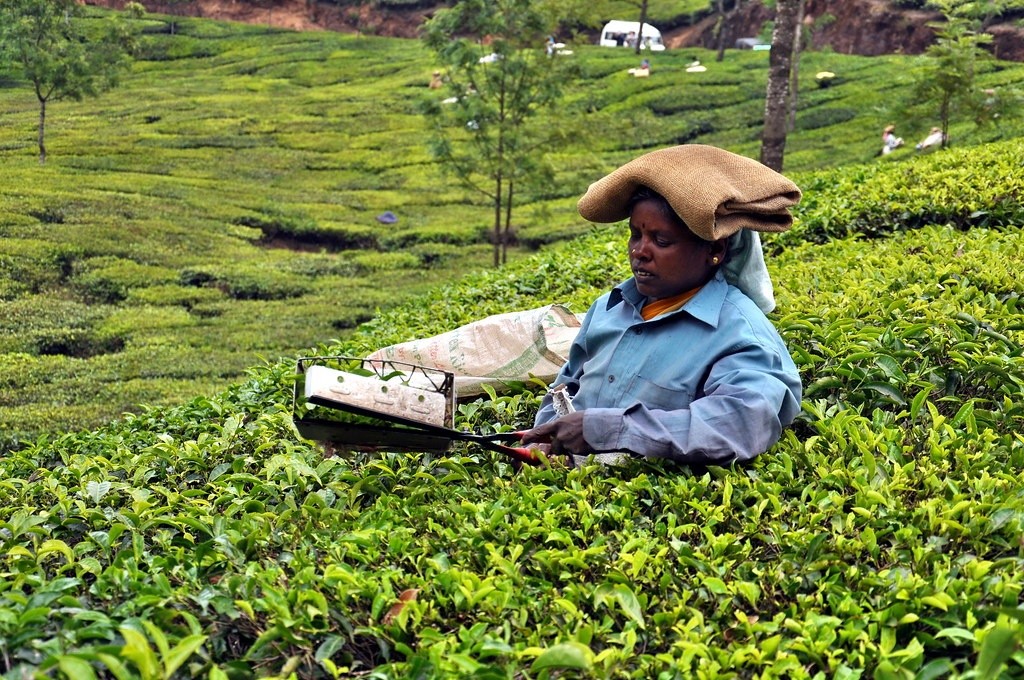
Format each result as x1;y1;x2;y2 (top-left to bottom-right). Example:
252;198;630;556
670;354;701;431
291;355;553;463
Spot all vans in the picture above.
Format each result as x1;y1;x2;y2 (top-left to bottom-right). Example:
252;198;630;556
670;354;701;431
600;20;665;51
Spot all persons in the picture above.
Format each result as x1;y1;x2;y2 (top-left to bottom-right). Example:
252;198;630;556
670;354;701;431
612;32;652;49
883;125;904;151
519;185;802;469
